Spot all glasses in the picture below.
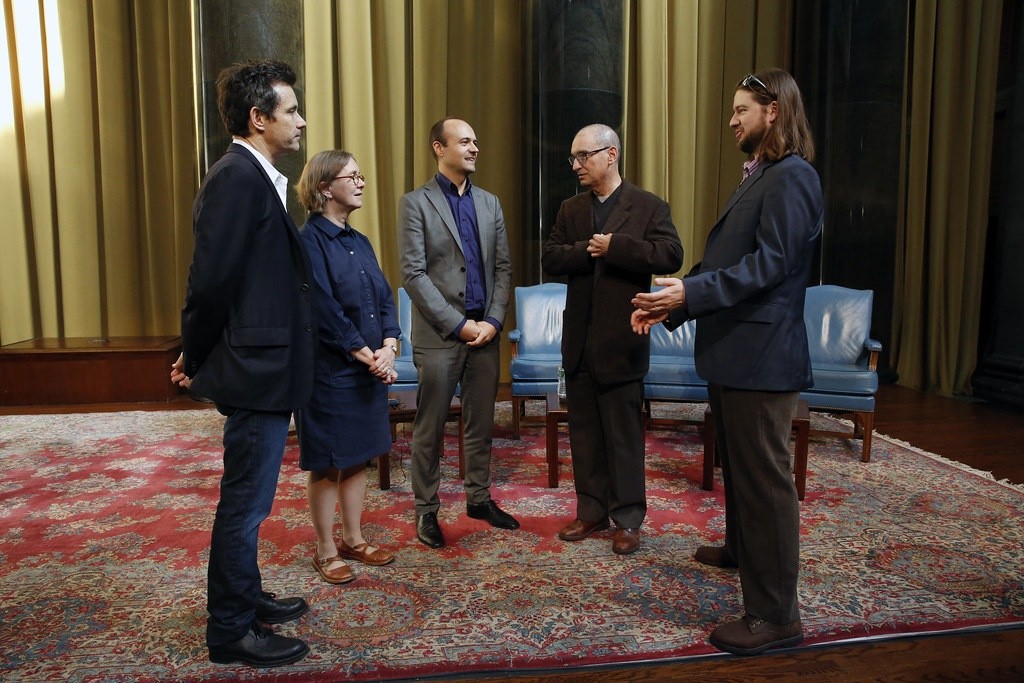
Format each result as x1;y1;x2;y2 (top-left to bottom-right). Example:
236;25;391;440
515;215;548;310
568;146;610;165
740;73;770;95
321;174;366;186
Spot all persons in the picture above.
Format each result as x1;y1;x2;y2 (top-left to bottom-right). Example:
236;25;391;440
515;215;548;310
168;59;310;666
629;68;824;656
543;122;686;554
399;117;522;548
289;148;402;584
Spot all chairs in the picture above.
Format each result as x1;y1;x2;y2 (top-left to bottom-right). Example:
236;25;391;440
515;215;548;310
378;287;466;441
508;282;571;440
643;286;723;468
795;285;884;462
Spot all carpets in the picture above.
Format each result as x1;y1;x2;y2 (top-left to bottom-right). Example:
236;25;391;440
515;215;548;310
0;398;1023;683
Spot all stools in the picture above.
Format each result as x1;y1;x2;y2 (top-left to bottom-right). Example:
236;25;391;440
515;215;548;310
705;398;810;500
546;392;648;488
378;391;466;489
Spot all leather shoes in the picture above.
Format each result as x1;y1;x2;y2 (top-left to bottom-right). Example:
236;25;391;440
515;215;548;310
414;511;446;549
613;525;641;552
695;542;737;569
208;624;310;668
252;592;309;623
466;498;521;530
558;515;611;541
709;610;803;654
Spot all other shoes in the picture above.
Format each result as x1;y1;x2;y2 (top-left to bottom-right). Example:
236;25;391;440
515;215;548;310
311;546;354;584
335;535;394;565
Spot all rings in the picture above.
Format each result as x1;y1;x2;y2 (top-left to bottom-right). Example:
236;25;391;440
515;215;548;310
383;368;387;372
656;306;663;312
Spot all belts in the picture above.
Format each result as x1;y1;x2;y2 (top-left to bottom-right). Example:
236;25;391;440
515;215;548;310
463;312;484;322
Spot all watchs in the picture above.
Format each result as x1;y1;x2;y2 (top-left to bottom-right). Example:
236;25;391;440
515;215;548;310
386;345;397;354
